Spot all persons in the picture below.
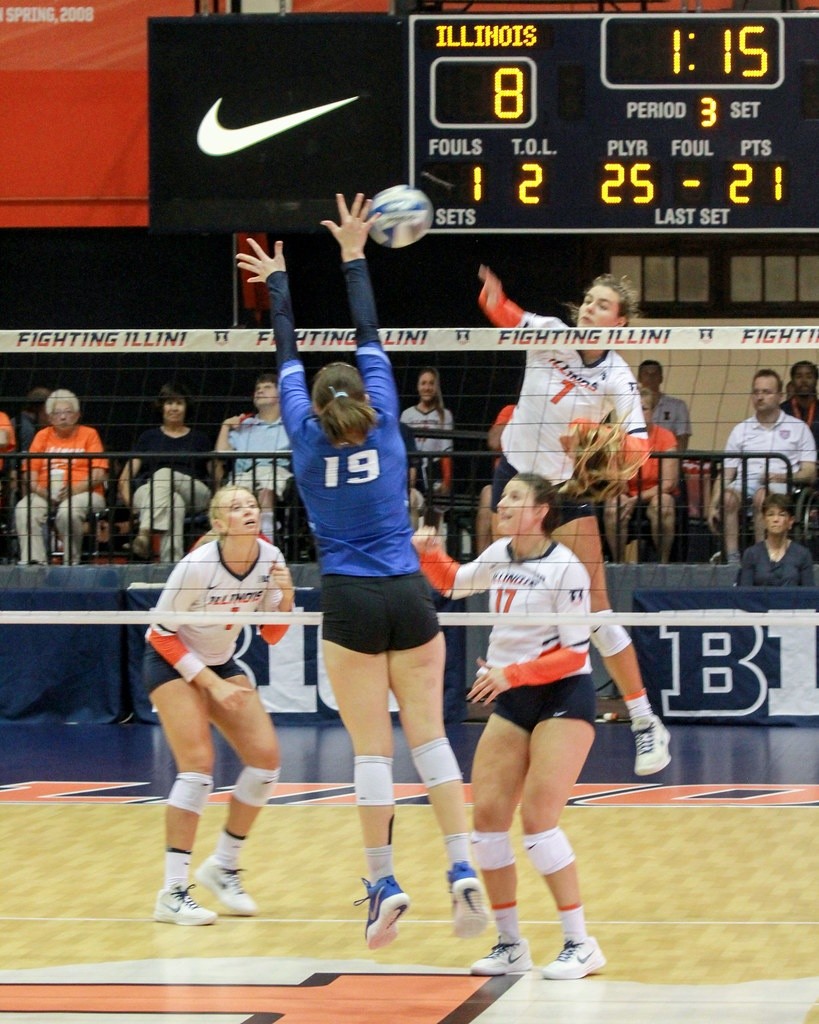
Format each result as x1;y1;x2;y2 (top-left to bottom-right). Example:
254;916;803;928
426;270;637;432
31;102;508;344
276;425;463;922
143;485;295;926
228;193;491;947
478;262;672;775
410;473;607;979
0;360;819;587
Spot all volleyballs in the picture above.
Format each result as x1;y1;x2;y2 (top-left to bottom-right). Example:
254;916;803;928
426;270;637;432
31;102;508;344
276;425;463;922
368;184;435;250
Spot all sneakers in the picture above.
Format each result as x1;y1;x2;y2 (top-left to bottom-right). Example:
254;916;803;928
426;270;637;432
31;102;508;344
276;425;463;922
353;873;408;949
153;882;216;927
630;713;670;776
449;861;488;938
470;937;533;976
194;856;257;918
543;935;605;979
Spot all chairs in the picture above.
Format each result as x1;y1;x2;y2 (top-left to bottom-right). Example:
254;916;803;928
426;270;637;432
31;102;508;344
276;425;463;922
0;441;819;556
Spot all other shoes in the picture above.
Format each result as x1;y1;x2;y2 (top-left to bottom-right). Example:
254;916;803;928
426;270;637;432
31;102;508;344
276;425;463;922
123;537;149;564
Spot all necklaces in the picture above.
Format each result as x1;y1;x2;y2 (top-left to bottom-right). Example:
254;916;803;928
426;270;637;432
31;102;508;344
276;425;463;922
768;541;789;556
